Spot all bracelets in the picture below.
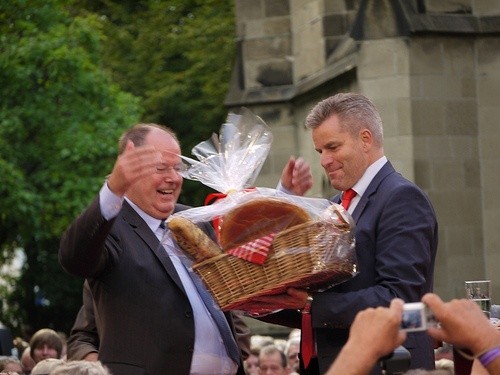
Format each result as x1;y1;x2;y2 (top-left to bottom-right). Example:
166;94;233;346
479;346;500;368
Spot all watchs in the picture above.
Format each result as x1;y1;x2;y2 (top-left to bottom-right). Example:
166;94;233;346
297;291;313;315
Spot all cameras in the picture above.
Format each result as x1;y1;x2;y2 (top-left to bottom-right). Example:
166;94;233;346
399;303;438;331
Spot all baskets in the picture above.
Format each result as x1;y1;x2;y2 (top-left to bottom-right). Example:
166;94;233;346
192;215;354;311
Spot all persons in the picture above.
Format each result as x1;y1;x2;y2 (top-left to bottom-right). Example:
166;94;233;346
58;122;313;375
244;92;440;375
0;279;500;375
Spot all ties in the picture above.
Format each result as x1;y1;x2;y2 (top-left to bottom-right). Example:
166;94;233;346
158;221;242;366
301;188;358;368
